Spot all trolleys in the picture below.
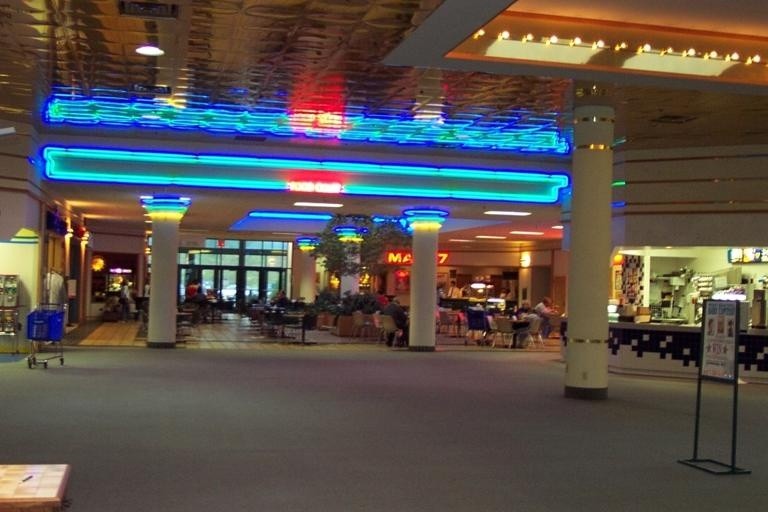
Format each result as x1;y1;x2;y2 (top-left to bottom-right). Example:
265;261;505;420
25;303;65;369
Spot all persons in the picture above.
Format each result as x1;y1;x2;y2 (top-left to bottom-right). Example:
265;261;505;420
510;297;560;338
185;279;205;312
270;289;288;307
384;297;409;347
118;277;132;324
206;287;219;304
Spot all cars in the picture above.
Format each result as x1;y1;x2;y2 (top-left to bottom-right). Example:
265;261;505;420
221;287;259;311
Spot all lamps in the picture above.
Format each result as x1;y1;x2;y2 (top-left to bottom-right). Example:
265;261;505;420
470;275;494;290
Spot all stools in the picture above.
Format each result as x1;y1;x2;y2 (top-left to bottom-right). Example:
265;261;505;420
119;300;130;324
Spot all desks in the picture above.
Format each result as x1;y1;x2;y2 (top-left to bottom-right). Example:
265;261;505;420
0;463;72;512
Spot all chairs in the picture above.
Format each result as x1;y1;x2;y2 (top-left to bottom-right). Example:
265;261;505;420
179;301;561;348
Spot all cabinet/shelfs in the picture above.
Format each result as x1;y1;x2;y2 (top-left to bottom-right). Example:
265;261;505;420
0;274;20;337
608;247;767;379
